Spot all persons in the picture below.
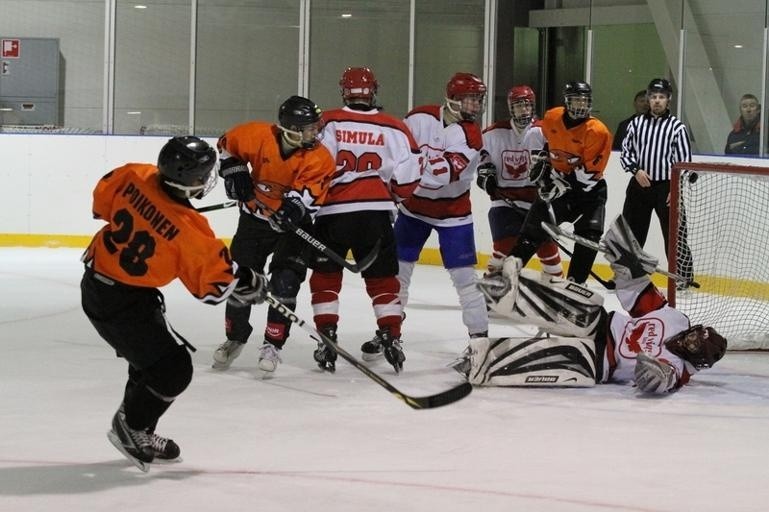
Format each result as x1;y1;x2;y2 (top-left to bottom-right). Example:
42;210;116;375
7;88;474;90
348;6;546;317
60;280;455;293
314;66;424;364
618;79;693;291
359;72;489;353
612;90;650;152
508;79;614;283
724;94;761;155
212;94;338;373
475;84;564;279
448;236;728;394
80;135;273;463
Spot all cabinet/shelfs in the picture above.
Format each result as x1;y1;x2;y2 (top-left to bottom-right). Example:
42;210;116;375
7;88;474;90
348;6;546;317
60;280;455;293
0;37;66;127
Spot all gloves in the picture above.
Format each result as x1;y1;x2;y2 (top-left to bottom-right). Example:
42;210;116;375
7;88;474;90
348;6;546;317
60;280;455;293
233;267;273;305
268;196;307;233
633;353;678;395
475;172;501;203
601;235;649;281
217;164;257;203
537;175;571;200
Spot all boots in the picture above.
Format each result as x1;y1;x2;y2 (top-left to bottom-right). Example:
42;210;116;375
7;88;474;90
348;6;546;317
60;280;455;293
379;331;407;373
314;323;338;372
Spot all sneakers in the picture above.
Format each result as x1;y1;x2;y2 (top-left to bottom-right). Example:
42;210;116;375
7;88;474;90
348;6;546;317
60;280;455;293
106;409;154;475
211;339;245;370
361;335;384;361
259;339;279;380
144;431;182;464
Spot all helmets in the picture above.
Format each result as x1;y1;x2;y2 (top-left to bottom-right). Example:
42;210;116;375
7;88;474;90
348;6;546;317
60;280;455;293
276;95;322;149
507;85;536;124
445;72;486;120
339;66;379;105
159;136;217;199
560;79;594;116
647;78;675;99
